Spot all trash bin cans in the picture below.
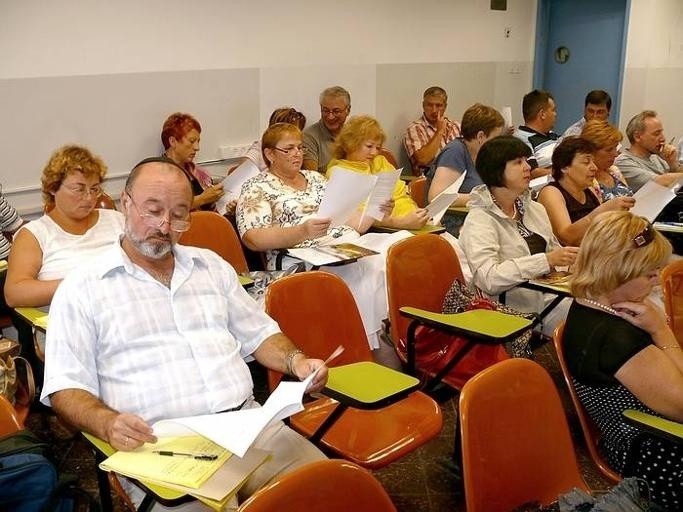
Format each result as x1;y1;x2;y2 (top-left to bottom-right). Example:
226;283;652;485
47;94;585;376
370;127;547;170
286;350;305;375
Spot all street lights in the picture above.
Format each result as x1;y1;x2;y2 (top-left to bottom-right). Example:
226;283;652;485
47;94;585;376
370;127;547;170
442;277;543;359
398;298;509;385
0;353;36;408
514;476;651;512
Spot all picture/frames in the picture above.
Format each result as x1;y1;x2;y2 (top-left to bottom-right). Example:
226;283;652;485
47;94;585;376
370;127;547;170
239;276;254;295
80;428;201;511
280;358;422;443
274;248;358;273
12;304;49;337
445;205;478;218
399;305;535;391
494;269;593;331
373;219;447;238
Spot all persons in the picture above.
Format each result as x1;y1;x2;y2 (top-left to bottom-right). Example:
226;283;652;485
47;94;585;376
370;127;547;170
162;113;238;217
539;137;635;245
561;213;683;511
554;91;612;149
429;105;504;208
303;87;351;171
457;135;580;313
325;115;442;230
402;87;463;176
513;91;562;179
0;188;42;383
580;120;635;200
236;122;395;271
614;111;683;197
4;146;124;309
41;158;330;512
227;108;306;177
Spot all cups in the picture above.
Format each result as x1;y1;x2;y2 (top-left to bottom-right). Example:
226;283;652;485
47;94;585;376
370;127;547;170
321;105;348;115
275;146;308;155
61;184;104;199
633;215;656;248
125;191;193;234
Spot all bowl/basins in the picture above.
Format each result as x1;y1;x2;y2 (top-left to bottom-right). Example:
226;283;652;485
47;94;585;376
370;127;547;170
581;297;617;315
492;195;517;219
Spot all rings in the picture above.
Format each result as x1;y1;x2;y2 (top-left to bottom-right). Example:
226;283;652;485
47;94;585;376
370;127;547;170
152;451;218;461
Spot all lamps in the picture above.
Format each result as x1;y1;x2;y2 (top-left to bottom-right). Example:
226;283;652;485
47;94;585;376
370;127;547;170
262;270;445;471
384;233;503;392
408;175;431;207
2;396;24;440
178;209;251;280
660;258;683;351
234;457;399;512
553;320;638;484
456;357;596;512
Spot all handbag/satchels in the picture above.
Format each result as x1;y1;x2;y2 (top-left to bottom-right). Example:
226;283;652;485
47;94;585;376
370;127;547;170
658;344;680;349
